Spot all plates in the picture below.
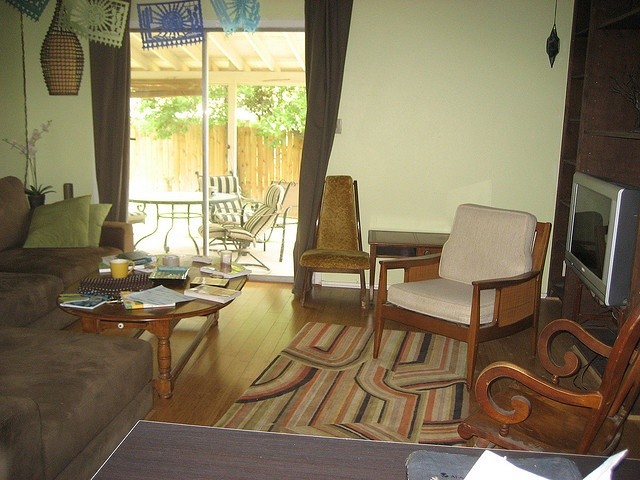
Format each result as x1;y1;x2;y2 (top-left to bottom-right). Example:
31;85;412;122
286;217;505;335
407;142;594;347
149;266;191;283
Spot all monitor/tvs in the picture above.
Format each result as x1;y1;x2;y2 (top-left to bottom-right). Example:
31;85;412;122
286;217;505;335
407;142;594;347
565;170;640;309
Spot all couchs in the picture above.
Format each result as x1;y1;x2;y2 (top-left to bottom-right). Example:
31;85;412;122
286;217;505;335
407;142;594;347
197;174;265;228
0;174;155;476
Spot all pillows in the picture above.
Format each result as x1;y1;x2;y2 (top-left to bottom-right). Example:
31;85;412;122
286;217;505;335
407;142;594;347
88;202;114;250
21;193;93;250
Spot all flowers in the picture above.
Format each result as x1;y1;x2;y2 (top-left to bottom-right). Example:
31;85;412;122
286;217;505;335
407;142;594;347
2;119;55;189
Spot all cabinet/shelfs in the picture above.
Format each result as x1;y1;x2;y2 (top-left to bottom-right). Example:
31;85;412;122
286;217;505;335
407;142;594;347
546;0;640;315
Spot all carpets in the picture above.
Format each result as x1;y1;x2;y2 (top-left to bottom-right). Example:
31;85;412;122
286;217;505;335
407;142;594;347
212;319;470;445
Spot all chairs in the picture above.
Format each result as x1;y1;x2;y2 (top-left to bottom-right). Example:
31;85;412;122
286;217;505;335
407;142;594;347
263;179;299;264
457;307;640;456
199;182;289;271
372;203;552;393
299;174;374;312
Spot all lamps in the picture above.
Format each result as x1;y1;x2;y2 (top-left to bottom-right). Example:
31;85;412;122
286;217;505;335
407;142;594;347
39;0;85;97
545;1;562;71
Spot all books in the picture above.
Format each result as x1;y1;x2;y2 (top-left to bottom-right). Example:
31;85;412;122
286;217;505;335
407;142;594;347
190;276;230;287
58;293;106;310
184;284;242;304
119;290;175;310
99;249;156;274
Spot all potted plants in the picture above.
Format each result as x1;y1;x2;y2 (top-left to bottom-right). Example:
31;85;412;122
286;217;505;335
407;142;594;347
23;181;56;210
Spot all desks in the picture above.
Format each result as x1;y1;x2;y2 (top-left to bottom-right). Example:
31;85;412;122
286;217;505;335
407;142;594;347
367;227;451;310
129;191;240;256
88;418;640;480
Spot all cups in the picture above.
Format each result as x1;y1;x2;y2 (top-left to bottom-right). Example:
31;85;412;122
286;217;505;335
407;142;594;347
221;251;232;266
110;258;135;278
